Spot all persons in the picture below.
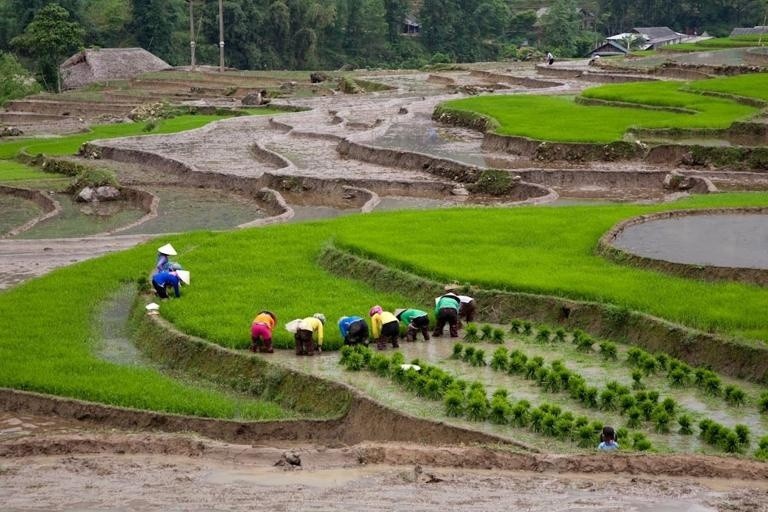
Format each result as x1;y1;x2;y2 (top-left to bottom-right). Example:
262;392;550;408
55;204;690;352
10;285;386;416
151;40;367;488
338;315;369;348
151;242;191;299
394;307;430;341
251;309;277;353
597;427;619;451
294;313;326;356
434;295;476;322
431;291;461;338
544;50;554;66
368;304;400;349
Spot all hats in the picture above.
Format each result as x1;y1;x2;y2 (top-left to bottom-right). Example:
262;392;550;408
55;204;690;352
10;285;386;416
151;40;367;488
442;290;461;304
176;270;191;285
393;308;408;317
257;310;276;326
434;296;443;307
157;243;177;256
